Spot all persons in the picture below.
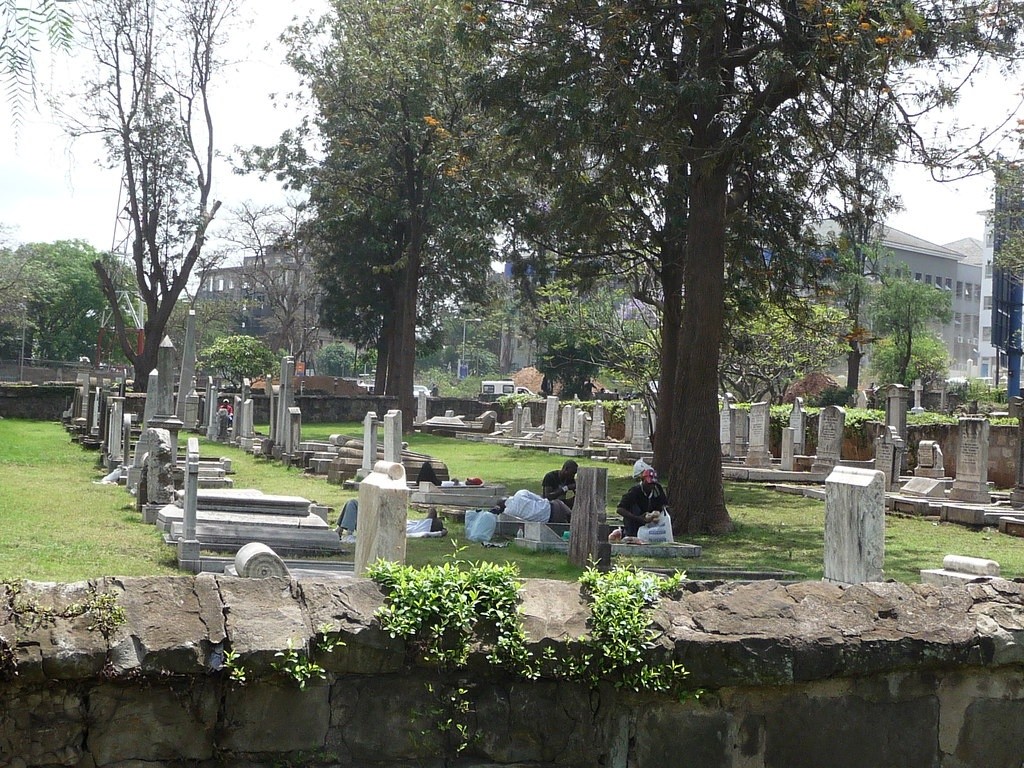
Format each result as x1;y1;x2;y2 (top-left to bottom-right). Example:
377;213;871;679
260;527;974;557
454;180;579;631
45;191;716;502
616;469;667;536
219;398;234;427
333;498;448;541
866;383;875;410
429;383;439;398
413;462;485;487
584;379;638;401
492;489;571;522
542;458;578;511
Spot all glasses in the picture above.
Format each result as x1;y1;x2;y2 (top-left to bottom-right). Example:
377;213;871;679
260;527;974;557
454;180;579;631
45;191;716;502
642;472;657;478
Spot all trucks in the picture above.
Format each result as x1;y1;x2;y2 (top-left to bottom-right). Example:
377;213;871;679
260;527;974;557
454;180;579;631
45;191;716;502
481;378;535;395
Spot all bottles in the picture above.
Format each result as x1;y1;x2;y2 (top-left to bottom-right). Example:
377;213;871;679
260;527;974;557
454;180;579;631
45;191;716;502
517;526;523;538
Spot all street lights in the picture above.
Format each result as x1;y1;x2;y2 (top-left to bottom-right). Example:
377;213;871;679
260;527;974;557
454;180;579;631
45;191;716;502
462;318;482;360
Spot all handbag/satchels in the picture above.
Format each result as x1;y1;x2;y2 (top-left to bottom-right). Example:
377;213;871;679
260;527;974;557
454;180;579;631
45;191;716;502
465;510;496;542
637;508;674;543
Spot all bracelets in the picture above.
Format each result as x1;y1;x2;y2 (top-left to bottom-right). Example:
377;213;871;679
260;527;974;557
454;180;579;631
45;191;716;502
562;486;568;492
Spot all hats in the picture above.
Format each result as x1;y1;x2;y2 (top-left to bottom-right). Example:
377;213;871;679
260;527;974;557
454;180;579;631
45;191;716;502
641;469;657;483
467;477;482;485
223;399;229;404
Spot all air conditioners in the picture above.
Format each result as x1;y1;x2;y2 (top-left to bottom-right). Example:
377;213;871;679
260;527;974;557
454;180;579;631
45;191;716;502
957;337;964;344
967;339;972;344
973;338;978;345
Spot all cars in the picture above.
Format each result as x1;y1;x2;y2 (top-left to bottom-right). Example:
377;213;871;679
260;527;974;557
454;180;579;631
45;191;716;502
412;385;432;399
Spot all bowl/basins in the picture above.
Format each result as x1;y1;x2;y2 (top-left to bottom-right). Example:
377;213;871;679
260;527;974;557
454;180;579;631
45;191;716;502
441;480;455;487
